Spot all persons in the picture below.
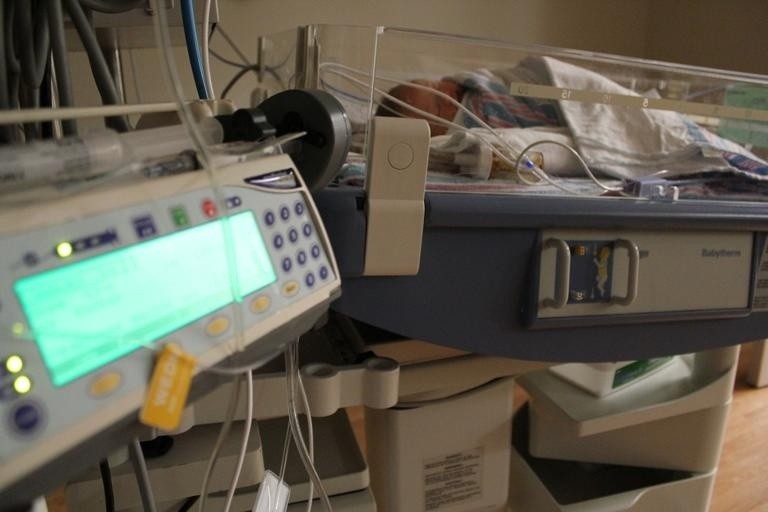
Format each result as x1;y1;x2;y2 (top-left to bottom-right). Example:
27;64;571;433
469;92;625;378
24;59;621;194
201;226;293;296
375;76;468;137
